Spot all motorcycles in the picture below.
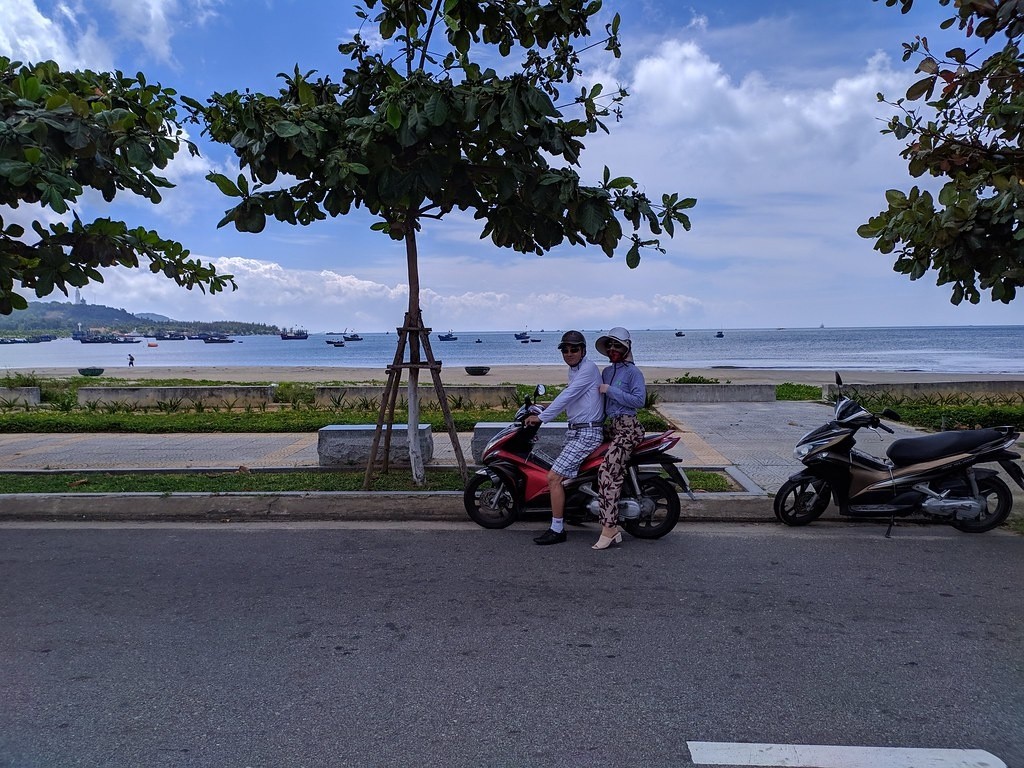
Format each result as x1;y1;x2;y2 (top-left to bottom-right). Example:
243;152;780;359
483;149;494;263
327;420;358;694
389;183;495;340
774;372;1024;539
464;385;697;539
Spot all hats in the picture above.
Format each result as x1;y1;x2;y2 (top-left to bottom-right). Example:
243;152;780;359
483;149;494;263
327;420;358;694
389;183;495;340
558;330;586;349
595;326;635;365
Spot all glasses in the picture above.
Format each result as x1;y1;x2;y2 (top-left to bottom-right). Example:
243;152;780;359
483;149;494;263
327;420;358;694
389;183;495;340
606;342;622;349
560;348;579;354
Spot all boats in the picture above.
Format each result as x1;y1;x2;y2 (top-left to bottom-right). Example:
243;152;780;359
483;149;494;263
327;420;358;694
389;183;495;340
188;333;235;344
280;324;309;339
514;325;530;339
0;334;57;344
326;328;347;335
437;330;458;341
343;333;363;341
325;339;345;347
716;332;724;338
675;332;685;337
144;332;186;340
531;339;542;342
72;329;143;344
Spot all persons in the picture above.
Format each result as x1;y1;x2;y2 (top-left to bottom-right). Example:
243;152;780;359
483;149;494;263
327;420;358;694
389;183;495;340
523;329;606;545
591;327;647;550
126;353;135;369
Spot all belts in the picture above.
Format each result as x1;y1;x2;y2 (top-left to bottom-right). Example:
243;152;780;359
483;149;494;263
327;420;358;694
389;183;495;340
568;422;603;430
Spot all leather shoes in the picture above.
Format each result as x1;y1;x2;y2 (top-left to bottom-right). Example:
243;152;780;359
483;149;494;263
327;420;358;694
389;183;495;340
533;528;567;545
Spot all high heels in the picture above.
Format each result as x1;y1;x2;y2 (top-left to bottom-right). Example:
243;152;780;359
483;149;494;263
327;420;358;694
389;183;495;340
592;532;622;550
